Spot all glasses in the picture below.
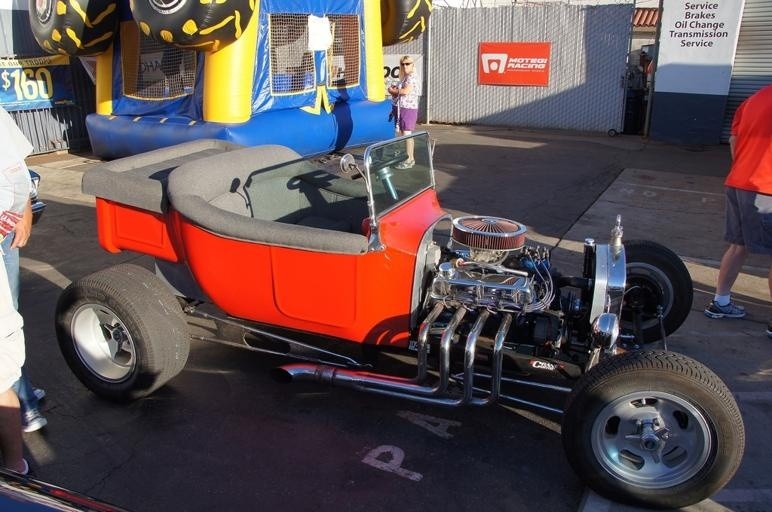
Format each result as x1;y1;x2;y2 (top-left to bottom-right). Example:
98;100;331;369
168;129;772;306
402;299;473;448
402;62;411;65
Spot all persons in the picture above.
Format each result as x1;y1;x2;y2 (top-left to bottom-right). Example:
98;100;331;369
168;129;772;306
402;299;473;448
0;252;30;475
704;82;772;338
388;55;421;170
0;104;46;433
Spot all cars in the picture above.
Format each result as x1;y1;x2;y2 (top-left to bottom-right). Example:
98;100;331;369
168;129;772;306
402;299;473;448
624;63;643;123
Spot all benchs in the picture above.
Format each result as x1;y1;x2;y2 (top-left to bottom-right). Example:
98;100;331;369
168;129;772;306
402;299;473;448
167;143;413;255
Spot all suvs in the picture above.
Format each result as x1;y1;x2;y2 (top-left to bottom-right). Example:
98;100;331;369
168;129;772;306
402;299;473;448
53;129;745;510
644;59;653;103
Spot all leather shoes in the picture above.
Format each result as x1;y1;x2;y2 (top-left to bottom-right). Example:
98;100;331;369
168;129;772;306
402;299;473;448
399;160;415;165
395;163;412;169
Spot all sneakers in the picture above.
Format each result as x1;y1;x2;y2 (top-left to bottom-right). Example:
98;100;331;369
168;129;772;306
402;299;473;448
20;409;49;432
704;300;747;320
32;386;45;400
